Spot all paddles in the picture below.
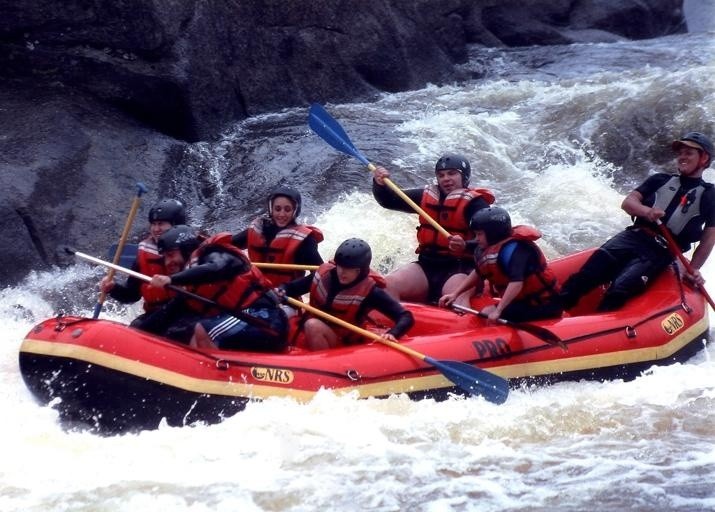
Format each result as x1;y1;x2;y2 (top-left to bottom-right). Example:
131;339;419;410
61;245;280;348
305;101;453;243
435;297;570;353
282;292;511;406
107;242;321;273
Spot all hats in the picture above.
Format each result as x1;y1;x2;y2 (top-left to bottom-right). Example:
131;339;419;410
671;139;704;153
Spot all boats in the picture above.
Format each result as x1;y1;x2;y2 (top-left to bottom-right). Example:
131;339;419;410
19;245;711;434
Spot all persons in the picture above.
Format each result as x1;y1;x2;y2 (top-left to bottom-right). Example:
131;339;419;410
559;132;714;312
438;207;566;323
136;225;289;353
230;187;325;320
274;238;414;351
100;199;210;345
372;153;495;309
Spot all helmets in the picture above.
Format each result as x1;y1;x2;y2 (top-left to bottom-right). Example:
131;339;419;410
472;208;513;239
435;155;471;182
336;239;371;266
268;183;301;219
158;223;200;261
681;131;714;164
149;200;186;228
